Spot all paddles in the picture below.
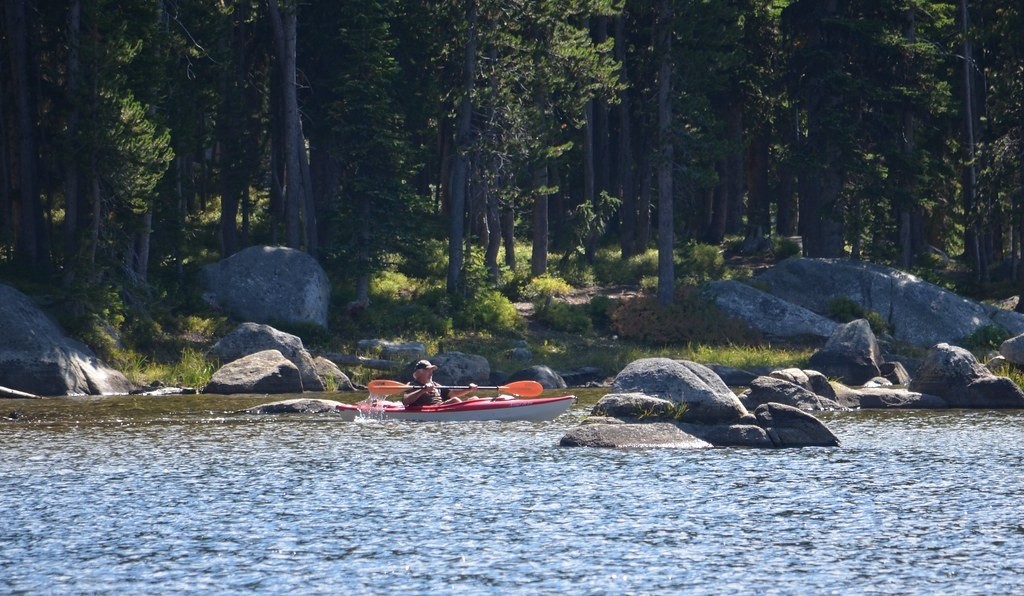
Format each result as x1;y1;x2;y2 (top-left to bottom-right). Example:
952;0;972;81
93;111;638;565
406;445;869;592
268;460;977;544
368;379;544;397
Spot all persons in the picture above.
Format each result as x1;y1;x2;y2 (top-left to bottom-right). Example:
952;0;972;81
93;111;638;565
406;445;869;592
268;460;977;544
402;360;478;408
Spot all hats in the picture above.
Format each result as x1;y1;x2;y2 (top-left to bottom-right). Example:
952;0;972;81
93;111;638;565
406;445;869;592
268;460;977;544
414;360;437;371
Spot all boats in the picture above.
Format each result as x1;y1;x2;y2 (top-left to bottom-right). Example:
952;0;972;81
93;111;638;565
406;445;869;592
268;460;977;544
333;394;577;422
